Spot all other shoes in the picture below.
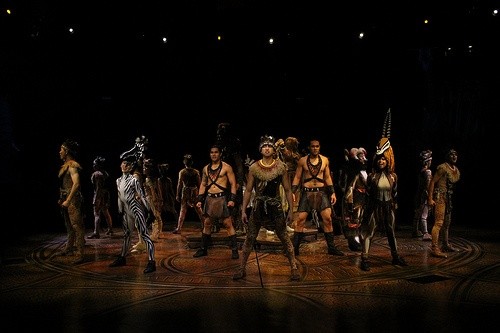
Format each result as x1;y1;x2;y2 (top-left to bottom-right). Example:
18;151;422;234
87;232;100;239
143;260;157;273
236;226;247;237
391;254;408;267
233;272;246;281
71;255;86;265
412;230;423;238
55;247;74;256
173;229;181;234
441;244;460;252
429;245;448;259
291;268;301;280
104;226;113;237
108;256;126;267
361;256;370;272
422;233;432;241
133;242;146;250
316;225;324;233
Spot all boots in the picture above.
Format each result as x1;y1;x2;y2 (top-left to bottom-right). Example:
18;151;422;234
229;234;239;259
324;232;348;256
348;236;363;252
193;231;211;257
284;231;302;257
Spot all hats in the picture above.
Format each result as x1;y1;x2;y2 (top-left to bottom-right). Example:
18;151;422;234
183;154;194;166
258;135;277;152
120;136;147;174
90;156;106;170
61;139;79;155
418;150;432;168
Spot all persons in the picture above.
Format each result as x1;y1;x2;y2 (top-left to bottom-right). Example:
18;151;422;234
233;134;299;281
58;141;239;274
243;139;462;273
